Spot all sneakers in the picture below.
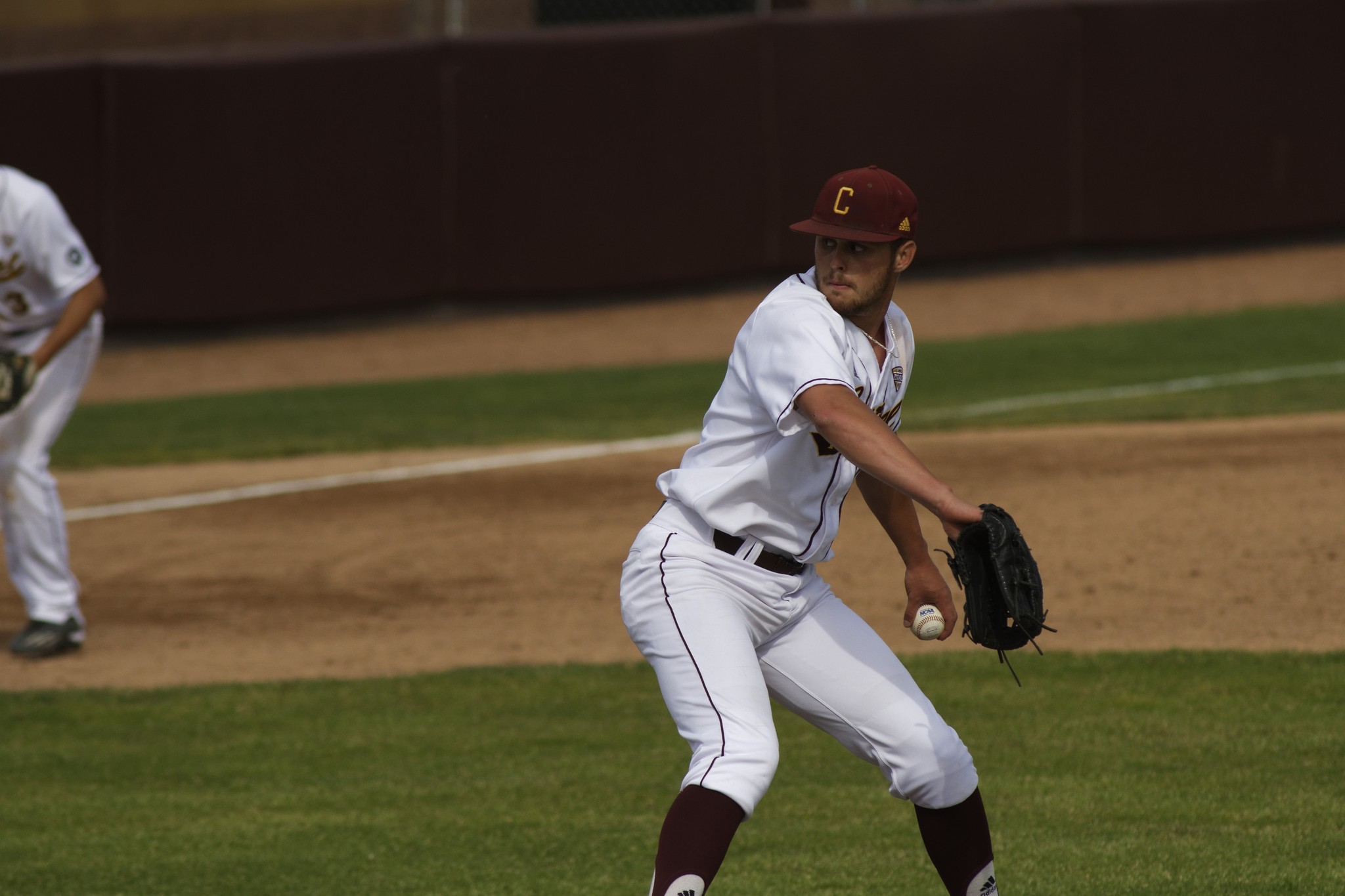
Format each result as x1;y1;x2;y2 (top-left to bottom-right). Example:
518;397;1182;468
9;617;84;656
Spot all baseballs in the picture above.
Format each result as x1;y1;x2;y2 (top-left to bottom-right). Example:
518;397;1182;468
909;603;946;641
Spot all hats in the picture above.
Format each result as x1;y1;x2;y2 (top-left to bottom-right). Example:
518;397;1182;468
789;165;919;242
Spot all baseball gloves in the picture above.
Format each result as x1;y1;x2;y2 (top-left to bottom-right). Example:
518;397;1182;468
947;502;1043;650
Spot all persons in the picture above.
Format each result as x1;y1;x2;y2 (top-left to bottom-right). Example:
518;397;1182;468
618;163;1015;895
0;163;107;657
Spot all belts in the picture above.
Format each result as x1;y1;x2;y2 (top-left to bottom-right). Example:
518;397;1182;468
713;528;807;577
